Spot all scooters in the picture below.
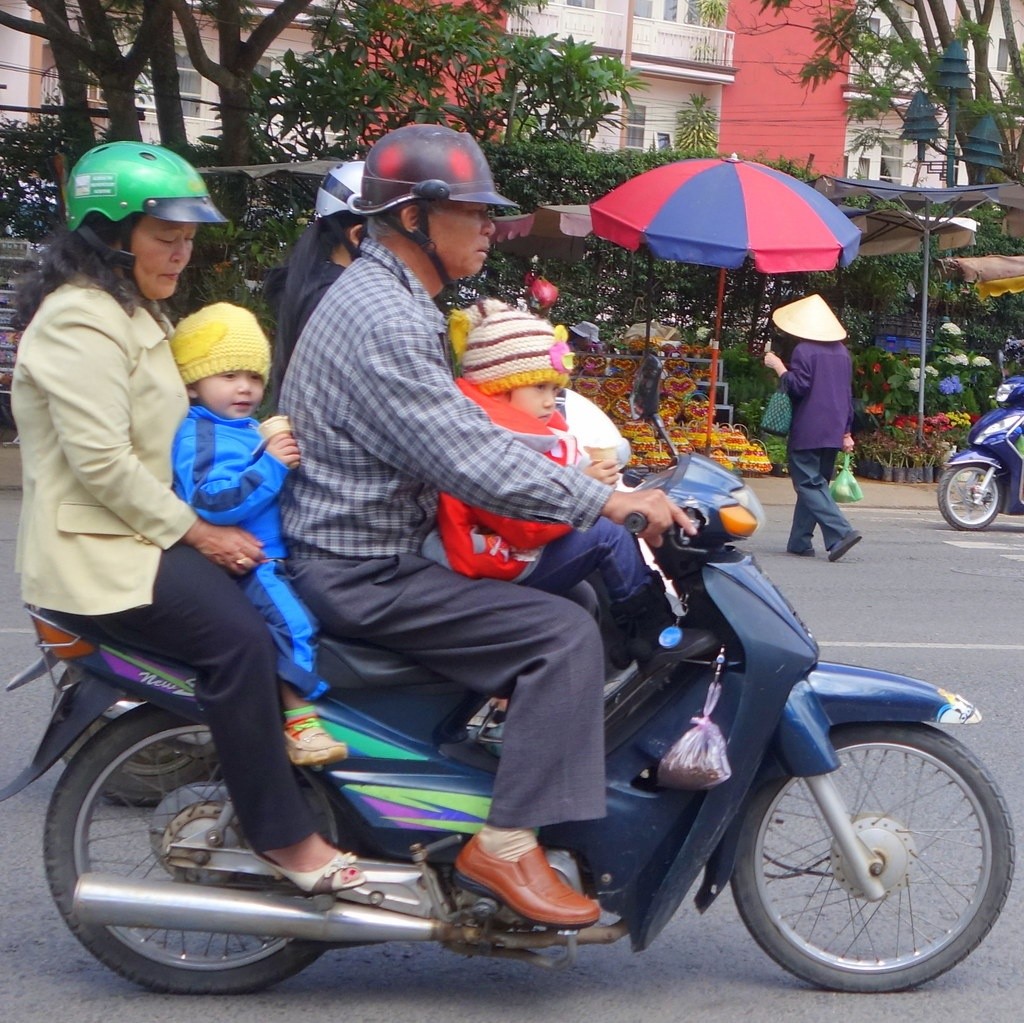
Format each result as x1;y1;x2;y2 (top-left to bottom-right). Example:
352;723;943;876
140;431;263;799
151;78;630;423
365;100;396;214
937;349;1024;533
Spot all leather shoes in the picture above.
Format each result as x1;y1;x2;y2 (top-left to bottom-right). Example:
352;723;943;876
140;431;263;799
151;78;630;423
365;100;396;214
452;827;600;928
252;850;366;893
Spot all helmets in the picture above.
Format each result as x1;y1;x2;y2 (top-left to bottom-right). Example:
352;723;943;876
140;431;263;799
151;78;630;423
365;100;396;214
65;141;230;232
316;161;365;216
347;124;519;214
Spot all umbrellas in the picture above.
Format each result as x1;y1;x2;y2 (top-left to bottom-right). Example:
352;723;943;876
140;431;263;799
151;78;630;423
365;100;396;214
804;173;1024;444
193;153;655;356
589;151;862;459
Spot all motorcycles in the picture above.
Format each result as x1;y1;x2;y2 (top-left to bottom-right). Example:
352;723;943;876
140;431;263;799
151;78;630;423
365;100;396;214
0;352;1016;996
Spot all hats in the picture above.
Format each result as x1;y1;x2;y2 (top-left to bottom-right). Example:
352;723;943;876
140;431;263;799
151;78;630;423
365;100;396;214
569;321;601;344
170;302;270;388
773;294;846;342
461;297;574;399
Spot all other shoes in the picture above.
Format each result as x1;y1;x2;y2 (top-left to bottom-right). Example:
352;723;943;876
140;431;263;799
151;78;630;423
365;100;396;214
800;549;815;557
828;530;862;561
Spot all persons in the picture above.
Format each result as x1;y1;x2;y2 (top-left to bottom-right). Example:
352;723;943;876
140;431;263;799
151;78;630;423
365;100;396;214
765;293;864;563
9;141;367;895
277;123;698;931
169;301;347;765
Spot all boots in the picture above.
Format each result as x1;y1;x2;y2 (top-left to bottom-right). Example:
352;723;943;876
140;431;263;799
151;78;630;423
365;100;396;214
609;571;721;679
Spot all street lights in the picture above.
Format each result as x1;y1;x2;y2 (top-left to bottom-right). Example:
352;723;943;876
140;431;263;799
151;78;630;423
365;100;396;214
898;39;1006;259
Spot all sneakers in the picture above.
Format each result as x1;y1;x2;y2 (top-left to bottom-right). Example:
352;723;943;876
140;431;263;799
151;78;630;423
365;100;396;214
284;730;346;766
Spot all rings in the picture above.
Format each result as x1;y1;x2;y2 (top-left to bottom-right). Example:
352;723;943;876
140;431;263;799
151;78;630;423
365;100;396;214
236;557;249;564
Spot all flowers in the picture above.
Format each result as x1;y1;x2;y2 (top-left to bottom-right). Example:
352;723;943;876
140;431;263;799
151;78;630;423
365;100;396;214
895;410;980;446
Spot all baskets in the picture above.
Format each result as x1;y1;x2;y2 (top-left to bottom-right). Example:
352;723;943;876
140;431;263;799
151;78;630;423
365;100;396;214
622;420;773;477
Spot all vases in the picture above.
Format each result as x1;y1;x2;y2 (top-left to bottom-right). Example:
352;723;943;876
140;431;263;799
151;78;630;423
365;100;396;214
943;444;957;456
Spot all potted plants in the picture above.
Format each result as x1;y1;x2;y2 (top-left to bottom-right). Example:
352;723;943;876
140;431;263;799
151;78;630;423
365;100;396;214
852;426;934;484
762;435;789;477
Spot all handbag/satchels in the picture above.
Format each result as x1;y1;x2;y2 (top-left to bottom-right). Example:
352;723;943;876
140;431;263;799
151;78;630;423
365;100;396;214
830;453;863;503
759;383;792;437
657;683;731;790
